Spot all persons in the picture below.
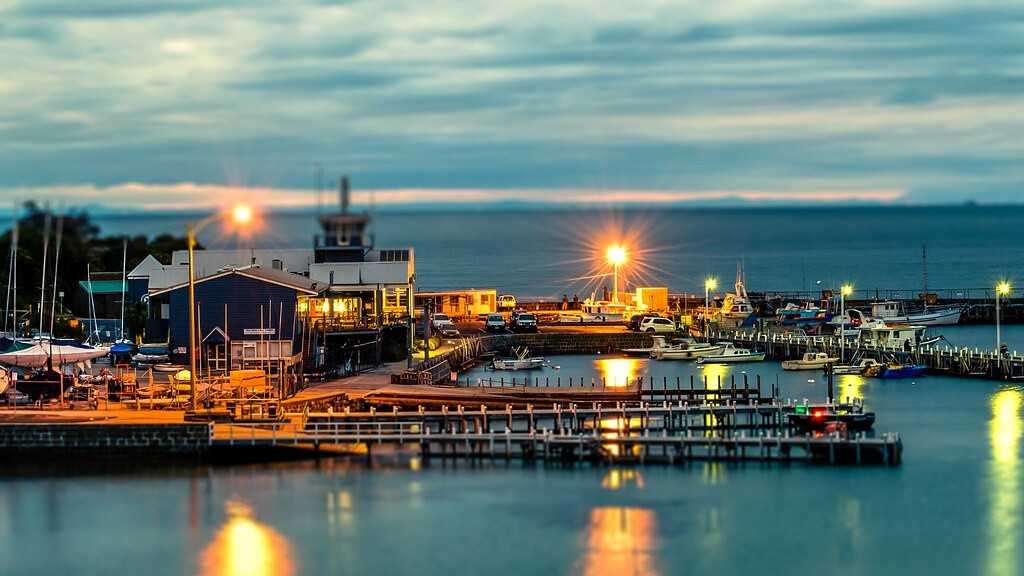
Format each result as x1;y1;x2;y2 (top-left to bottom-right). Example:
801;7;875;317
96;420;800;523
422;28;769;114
904;338;910;351
1000;342;1009;358
562;295;568;310
573;294;579;310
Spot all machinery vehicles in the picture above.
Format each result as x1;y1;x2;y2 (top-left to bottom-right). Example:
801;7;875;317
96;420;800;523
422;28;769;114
107;365;140;403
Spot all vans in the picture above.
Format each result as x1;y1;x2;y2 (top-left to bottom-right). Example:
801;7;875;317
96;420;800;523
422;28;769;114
497;295;515;310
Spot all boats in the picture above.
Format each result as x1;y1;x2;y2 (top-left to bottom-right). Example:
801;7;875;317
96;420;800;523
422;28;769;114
492;359;546;370
716;264;836;334
623;335;765;363
781;352;839;370
832;350;926;379
868;302;963;325
826;308;944;351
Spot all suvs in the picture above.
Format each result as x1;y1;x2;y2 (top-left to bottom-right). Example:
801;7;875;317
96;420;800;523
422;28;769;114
625;313;675;333
515;313;537;332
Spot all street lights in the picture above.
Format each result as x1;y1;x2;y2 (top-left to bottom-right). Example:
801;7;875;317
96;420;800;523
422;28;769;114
705;280;714;322
609;248;621;303
188;206;249;411
995;285;1006;367
841;285;850;362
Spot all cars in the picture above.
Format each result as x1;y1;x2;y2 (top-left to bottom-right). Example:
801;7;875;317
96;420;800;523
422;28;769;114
431;313;453;328
486;313;506;331
438;324;460;339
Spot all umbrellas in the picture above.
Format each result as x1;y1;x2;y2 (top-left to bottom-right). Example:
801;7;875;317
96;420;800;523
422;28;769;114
110;343;132;352
